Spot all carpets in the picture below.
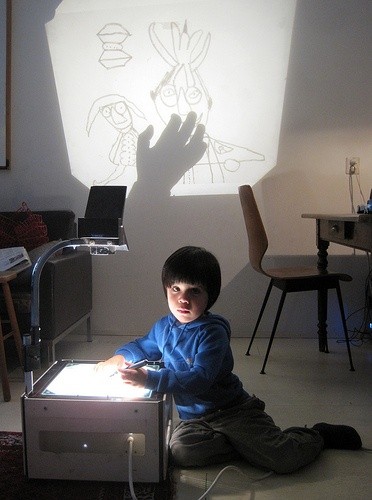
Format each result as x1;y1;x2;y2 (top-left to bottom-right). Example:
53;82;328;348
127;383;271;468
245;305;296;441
0;430;177;500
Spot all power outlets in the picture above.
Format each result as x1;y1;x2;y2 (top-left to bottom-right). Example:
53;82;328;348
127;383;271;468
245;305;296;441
346;158;359;174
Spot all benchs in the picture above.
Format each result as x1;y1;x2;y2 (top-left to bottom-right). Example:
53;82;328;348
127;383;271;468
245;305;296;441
0;210;92;369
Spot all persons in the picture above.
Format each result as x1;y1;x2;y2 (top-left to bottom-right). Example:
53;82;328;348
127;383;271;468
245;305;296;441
99;245;363;473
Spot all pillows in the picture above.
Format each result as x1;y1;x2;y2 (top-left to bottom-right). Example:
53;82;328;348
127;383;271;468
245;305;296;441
16;238;62;284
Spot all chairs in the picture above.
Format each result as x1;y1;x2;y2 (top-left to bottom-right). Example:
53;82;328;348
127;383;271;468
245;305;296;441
238;185;354;375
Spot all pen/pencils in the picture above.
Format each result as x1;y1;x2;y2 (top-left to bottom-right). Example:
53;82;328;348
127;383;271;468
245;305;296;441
111;359;150;377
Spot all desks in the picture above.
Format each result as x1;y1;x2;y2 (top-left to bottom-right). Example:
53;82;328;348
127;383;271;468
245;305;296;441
0;272;23;402
301;214;372;353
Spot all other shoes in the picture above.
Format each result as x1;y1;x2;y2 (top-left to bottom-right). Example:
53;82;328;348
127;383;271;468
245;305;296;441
312;422;363;451
